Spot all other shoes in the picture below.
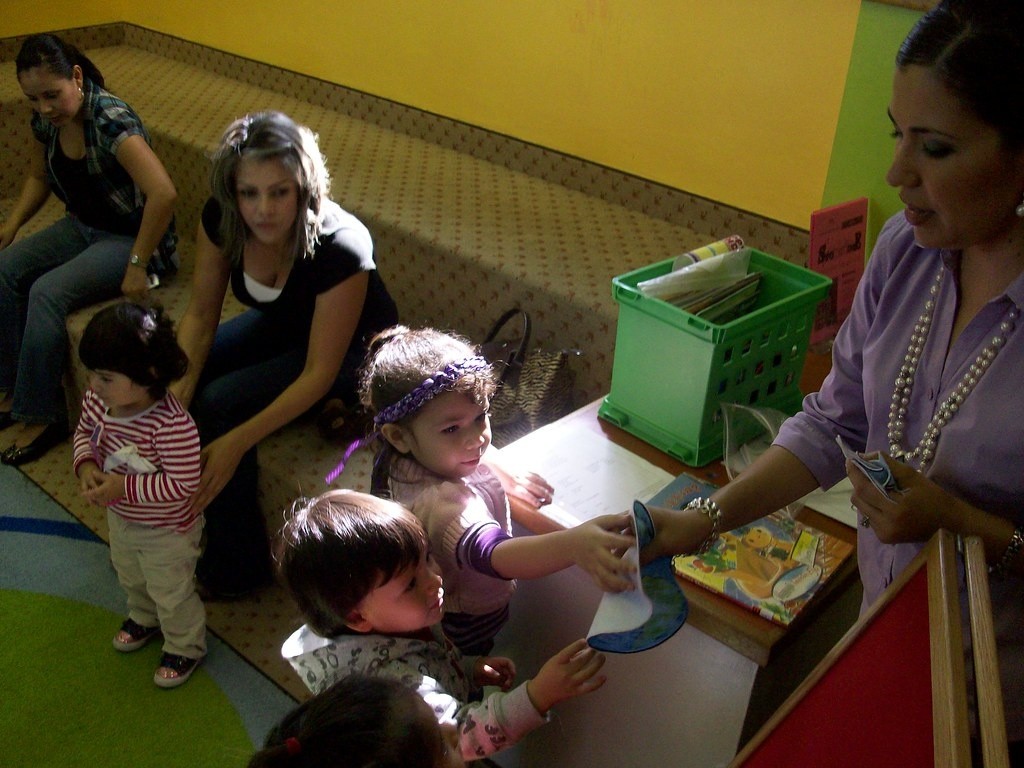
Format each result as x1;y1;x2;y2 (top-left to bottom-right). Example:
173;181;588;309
0;402;22;430
1;419;70;466
193;558;275;604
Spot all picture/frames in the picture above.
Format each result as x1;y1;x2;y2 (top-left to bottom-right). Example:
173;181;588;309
726;529;969;768
963;535;1024;768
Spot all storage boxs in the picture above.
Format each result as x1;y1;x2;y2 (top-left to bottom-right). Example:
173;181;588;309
598;243;832;466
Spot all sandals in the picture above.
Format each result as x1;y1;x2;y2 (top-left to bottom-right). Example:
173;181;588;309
112;619;160;652
154;652;199;687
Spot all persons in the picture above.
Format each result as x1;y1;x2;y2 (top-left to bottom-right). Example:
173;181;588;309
613;0;1024;619
168;112;399;600
248;674;464;768
277;489;608;768
73;303;208;687
0;33;180;464
362;325;637;705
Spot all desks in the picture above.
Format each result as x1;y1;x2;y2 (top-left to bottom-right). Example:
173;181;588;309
479;338;860;768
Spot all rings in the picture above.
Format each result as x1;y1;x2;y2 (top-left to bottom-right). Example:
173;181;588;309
862;515;870;528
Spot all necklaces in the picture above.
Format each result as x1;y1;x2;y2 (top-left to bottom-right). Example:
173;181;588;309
887;267;1020;474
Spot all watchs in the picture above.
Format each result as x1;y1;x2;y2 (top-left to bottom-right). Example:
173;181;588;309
128;255;147;268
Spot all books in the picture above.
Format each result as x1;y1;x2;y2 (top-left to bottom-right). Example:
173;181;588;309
646;473;855;629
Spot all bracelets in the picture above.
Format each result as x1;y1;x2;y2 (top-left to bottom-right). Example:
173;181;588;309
684;497;724;557
994;525;1024;579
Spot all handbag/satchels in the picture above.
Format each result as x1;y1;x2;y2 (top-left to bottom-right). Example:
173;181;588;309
477;308;570;450
